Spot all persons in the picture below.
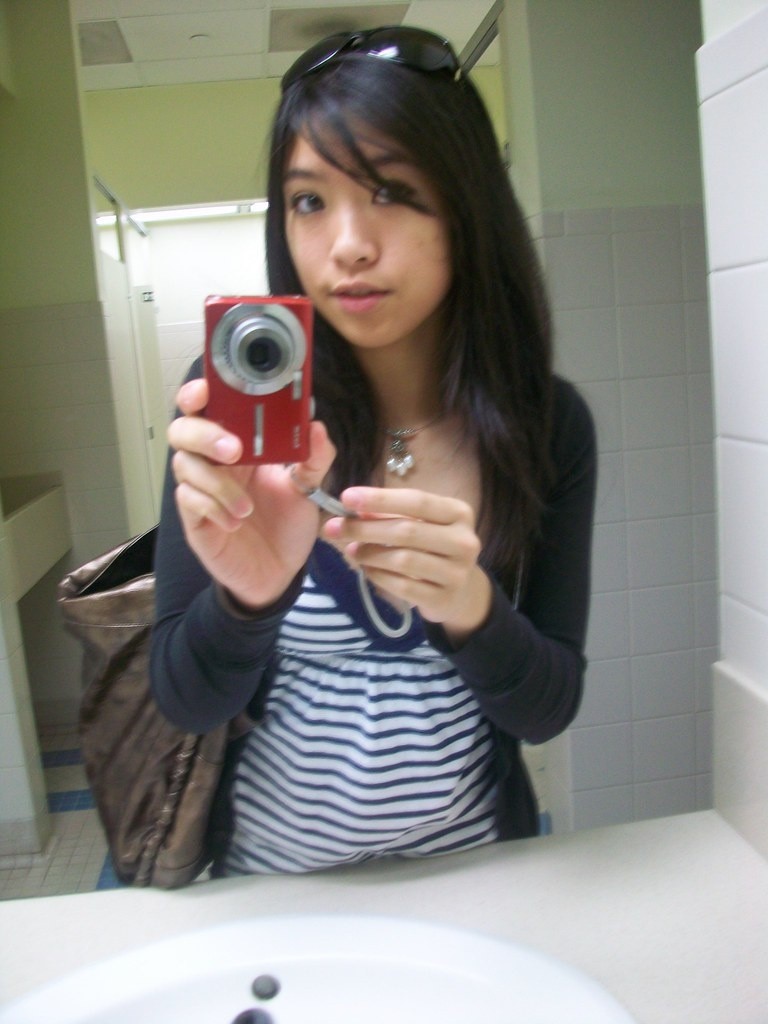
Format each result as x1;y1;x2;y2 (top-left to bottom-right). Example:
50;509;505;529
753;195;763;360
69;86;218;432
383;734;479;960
151;28;598;868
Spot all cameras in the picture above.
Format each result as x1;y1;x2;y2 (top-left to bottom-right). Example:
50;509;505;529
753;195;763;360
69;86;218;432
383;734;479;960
204;295;314;468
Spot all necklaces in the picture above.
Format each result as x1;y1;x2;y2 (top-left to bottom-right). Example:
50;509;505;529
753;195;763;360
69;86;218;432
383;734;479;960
374;413;440;477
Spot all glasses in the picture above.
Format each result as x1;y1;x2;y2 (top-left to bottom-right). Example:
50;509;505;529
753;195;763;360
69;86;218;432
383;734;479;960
280;26;465;94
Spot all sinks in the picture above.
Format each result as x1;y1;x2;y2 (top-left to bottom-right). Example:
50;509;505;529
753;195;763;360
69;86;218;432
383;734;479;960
0;909;650;1024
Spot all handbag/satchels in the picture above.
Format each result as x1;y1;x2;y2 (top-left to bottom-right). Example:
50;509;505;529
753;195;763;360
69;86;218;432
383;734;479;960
57;521;268;889
